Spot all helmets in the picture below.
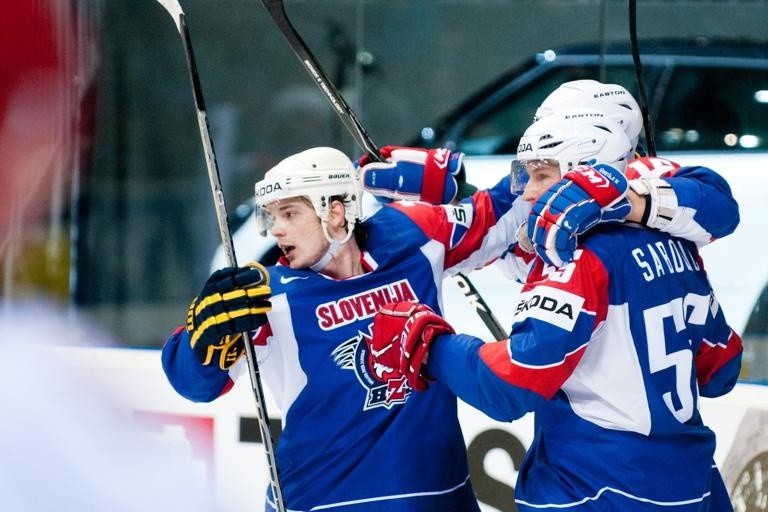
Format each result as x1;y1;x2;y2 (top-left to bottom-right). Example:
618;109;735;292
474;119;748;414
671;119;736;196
253;147;362;245
511;80;642;199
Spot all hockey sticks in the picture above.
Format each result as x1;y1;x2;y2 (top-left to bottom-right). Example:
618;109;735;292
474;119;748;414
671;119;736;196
159;0;284;512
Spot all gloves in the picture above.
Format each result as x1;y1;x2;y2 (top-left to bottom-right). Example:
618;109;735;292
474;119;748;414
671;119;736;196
185;268;271;371
527;165;632;268
372;301;455;392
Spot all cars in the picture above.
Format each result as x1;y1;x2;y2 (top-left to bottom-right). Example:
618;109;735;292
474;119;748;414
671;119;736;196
205;35;768;382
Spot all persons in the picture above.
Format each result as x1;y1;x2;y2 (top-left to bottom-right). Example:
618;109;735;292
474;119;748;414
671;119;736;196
156;160;537;510
